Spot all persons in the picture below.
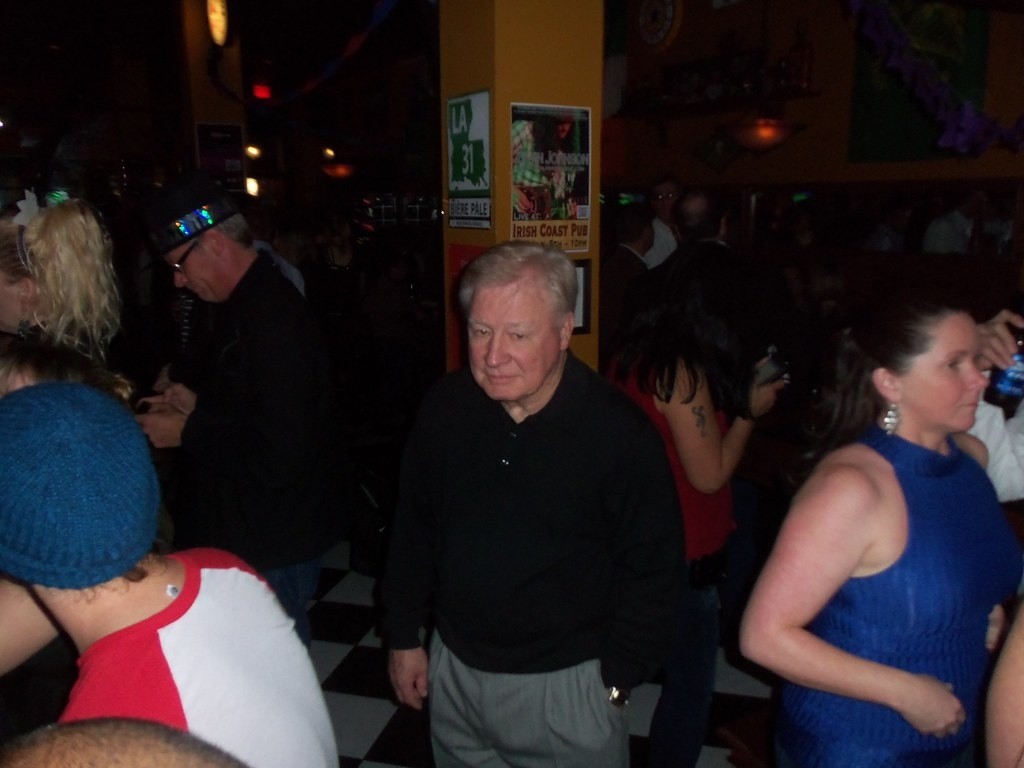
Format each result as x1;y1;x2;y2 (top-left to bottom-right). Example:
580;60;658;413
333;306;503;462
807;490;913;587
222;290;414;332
510;107;586;218
0;177;1024;768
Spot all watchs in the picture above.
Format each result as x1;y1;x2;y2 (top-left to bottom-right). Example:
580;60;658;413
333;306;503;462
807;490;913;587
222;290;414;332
609;685;629;705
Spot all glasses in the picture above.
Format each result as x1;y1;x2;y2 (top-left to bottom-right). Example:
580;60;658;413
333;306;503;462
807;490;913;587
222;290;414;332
164;241;199;275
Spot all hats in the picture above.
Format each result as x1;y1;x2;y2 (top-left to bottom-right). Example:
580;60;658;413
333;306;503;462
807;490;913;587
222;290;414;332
0;380;161;589
153;177;240;255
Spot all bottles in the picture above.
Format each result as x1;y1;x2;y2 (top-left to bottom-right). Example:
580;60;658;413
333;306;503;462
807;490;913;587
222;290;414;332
984;291;1024;409
